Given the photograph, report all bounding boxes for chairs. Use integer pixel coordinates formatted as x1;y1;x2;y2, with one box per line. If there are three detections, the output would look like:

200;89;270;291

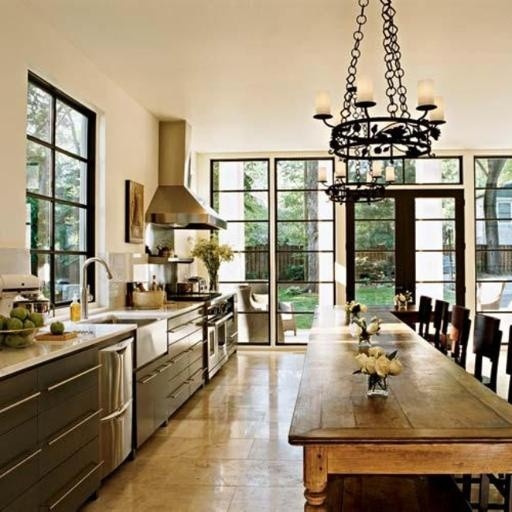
415;295;512;404
456;279;505;317
240;285;296;342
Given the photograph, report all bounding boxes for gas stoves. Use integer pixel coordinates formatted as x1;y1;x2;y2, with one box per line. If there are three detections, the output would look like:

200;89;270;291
166;291;222;301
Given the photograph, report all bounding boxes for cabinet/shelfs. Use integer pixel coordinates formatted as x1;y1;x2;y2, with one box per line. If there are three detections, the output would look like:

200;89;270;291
1;346;101;512
206;292;238;380
136;300;206;451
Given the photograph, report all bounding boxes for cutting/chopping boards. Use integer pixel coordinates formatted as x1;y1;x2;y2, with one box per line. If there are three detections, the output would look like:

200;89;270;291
35;333;78;341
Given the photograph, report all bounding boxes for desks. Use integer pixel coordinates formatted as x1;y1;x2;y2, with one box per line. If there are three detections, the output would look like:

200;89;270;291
291;302;512;511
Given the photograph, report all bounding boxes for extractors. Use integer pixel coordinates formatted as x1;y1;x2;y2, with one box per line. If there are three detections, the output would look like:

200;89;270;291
145;120;227;231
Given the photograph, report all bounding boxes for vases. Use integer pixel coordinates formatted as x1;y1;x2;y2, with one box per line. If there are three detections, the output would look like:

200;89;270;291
205;263;221;293
350;312;360;320
364;371;390;397
359;333;371;345
397;301;401;311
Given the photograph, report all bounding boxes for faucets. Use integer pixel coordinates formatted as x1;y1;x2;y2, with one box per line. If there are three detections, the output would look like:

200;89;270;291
81;257;113;319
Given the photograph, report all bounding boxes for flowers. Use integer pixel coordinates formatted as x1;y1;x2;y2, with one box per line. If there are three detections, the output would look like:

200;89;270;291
348;314;382;338
189;231;236;280
357;344;403;385
392;291;406;305
344;300;367;313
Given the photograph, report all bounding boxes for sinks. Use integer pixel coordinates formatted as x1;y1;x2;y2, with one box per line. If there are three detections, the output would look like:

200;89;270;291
74;313;158;331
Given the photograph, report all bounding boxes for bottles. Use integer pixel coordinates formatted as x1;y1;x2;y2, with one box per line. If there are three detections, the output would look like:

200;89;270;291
70;294;80;320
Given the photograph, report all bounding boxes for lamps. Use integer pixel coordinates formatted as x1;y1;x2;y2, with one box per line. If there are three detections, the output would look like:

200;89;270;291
313;0;447;205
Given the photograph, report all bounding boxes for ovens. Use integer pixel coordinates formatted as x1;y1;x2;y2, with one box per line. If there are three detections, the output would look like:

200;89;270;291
208;312;230;379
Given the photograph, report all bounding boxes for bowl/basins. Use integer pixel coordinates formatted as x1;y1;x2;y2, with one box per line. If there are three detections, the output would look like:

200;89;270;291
1;326;39;349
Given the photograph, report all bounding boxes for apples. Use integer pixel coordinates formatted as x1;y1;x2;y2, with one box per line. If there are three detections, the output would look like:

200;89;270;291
0;306;43;347
51;322;63;334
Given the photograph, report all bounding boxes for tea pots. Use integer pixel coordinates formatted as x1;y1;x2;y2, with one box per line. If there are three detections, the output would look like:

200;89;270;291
157;244;175;258
